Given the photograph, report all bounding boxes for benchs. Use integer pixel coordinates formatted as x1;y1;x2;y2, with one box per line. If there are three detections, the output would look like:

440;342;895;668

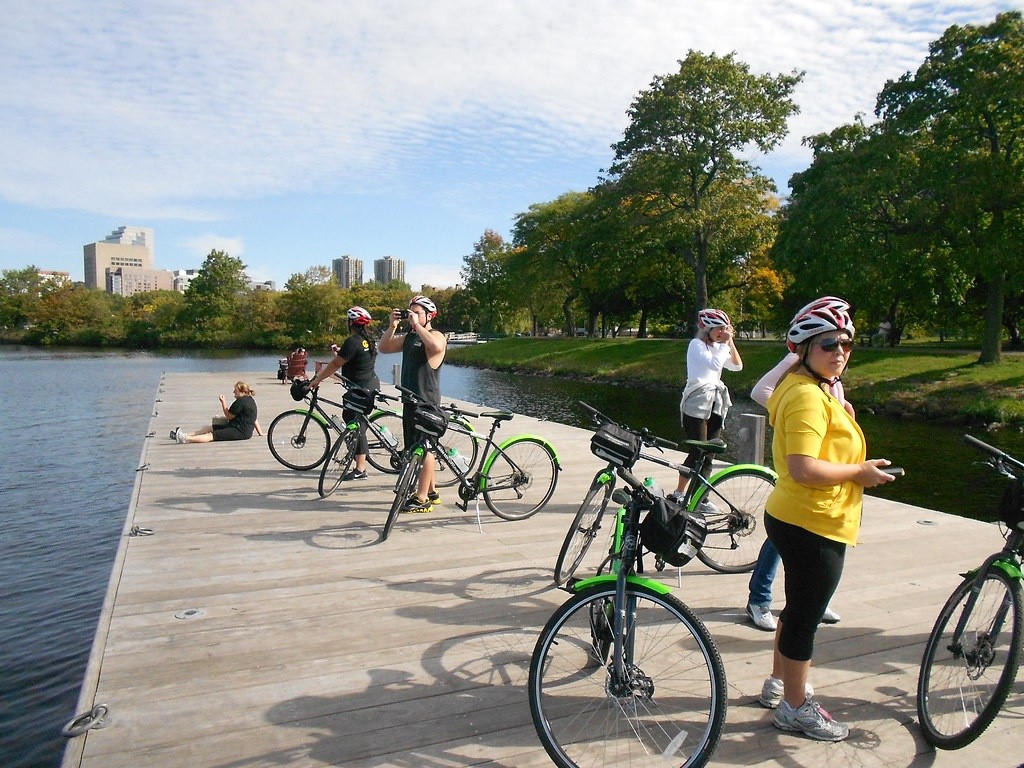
859;335;872;346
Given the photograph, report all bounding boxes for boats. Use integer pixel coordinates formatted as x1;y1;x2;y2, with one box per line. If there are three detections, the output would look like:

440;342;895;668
444;331;481;345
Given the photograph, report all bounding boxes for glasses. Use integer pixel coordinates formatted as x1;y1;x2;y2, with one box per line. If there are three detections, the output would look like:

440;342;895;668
809;337;854;354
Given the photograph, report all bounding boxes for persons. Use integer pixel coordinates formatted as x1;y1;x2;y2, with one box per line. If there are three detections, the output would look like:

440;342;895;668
673;309;743;513
305;306;380;481
170;382;267;443
759;307;895;741
745;296;855;630
872;317;891;347
379;295;446;513
293;348;308;364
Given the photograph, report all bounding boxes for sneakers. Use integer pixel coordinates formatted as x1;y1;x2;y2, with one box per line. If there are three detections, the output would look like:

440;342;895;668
773;695;851;742
408;488;441;505
744;602;777;631
758;674;815;710
169;430;177;440
399;495;434;514
341;467;368;481
675;488;687;506
821;608;841;626
698;500;724;515
175;426;187;444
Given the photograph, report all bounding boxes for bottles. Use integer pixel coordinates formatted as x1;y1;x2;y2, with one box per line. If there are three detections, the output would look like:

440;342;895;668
332;414;346;432
379;426;398;446
642;475;664;500
446;447;469;473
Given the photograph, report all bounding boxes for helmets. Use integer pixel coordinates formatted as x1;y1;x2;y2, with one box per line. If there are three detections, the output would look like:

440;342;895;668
408;295;437;318
786;295;854;326
345;305;373;326
697;308;731;329
786;306;856;354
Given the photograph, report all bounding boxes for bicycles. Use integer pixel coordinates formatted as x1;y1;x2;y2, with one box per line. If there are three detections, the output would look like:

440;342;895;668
380;384;563;542
267;372;408;475
527;463;729;768
916;433;1024;751
553;400;779;584
318;372;483;498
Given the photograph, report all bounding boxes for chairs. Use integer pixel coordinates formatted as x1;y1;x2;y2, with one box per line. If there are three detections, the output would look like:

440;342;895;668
283;354;309;384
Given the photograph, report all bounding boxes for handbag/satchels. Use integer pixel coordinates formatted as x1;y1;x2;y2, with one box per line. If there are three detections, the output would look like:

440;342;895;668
212;415;228;426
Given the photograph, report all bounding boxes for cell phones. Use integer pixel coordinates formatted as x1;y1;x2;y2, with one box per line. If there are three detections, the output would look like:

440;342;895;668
881;467;906;476
397;310;408;320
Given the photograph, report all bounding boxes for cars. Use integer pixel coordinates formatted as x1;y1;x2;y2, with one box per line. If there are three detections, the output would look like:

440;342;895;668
575;330;587;339
